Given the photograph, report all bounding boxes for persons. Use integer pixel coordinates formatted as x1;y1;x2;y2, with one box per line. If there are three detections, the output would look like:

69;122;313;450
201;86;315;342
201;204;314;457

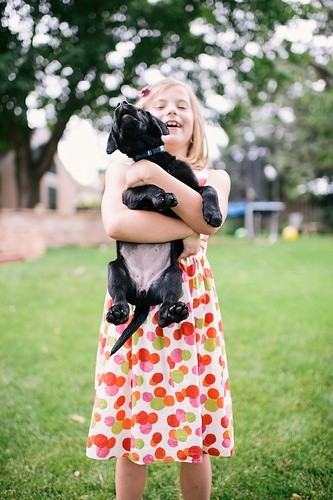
85;80;235;500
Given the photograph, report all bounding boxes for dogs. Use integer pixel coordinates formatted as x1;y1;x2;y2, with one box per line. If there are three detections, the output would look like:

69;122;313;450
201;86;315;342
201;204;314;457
106;100;223;358
